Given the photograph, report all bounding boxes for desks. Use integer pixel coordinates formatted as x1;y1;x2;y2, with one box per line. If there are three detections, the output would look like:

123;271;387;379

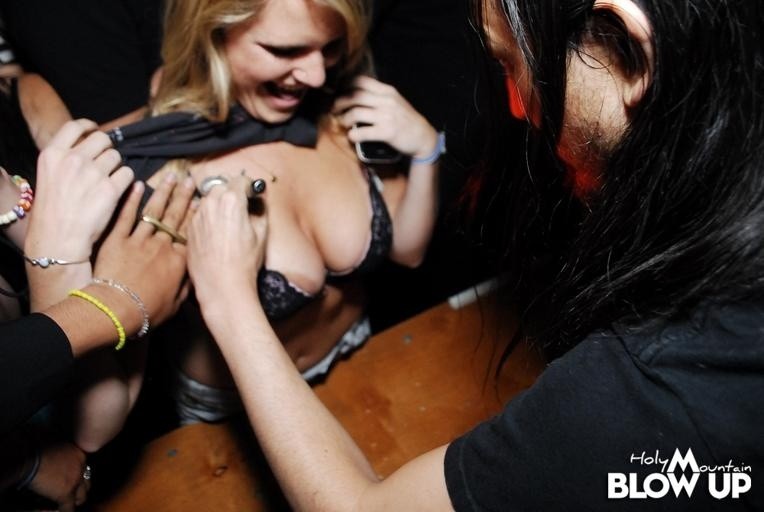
82;294;546;511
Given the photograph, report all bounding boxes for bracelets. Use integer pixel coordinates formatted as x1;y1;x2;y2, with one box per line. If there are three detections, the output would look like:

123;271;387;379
412;130;447;169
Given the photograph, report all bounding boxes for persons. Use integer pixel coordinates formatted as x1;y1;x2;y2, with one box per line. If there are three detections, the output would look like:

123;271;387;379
182;0;764;511
18;2;442;457
